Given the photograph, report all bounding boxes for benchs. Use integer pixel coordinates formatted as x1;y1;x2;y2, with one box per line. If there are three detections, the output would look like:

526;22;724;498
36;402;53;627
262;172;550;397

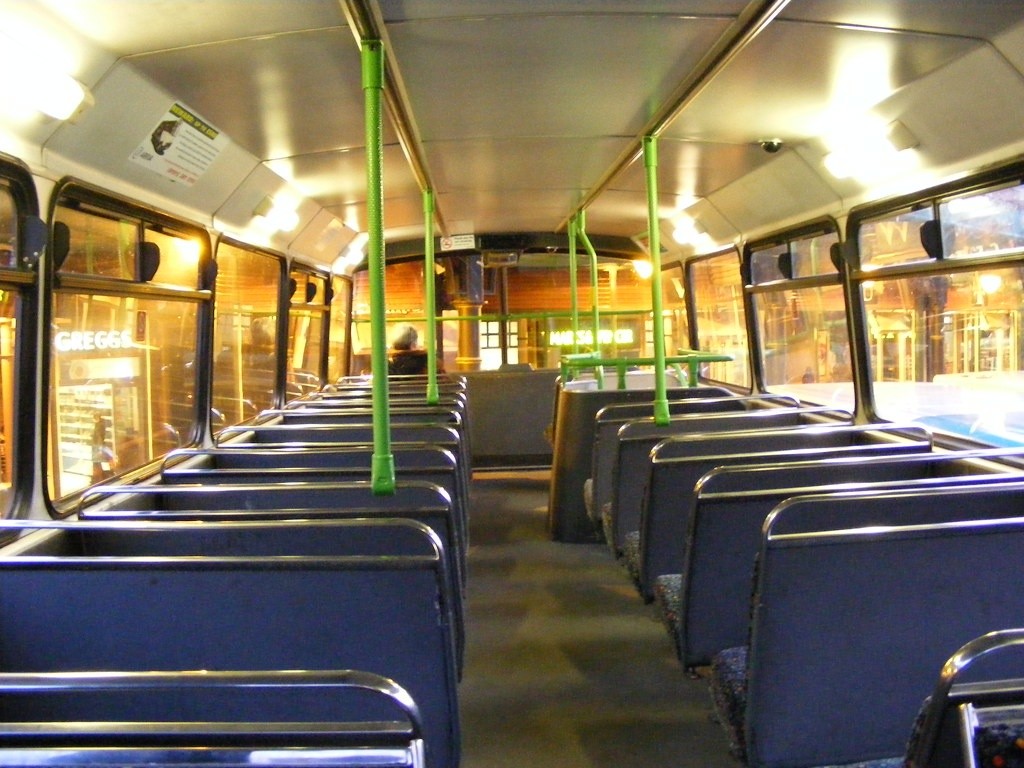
56;360;319;492
541;371;680;451
0;371;474;768
584;394;1024;768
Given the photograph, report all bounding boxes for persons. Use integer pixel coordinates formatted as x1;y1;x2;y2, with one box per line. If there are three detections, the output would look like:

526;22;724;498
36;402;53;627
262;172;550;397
387;323;445;375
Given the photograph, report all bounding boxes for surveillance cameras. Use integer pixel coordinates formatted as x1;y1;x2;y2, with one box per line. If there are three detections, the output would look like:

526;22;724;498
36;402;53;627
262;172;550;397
758;139;782;154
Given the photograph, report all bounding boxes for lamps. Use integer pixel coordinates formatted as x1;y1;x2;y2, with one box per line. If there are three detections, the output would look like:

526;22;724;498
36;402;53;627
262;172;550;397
37;79;95;127
255;196;300;232
821;118;921;180
